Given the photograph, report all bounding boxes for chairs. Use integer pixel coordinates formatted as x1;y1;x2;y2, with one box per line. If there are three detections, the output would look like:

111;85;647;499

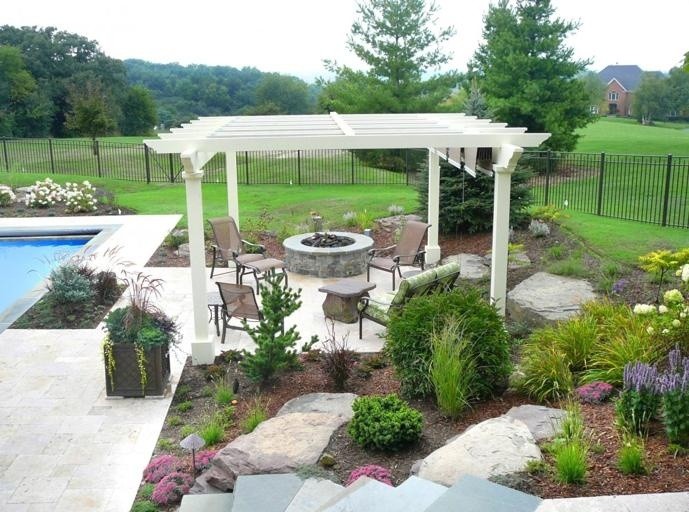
207;216;290;344
365;220;433;291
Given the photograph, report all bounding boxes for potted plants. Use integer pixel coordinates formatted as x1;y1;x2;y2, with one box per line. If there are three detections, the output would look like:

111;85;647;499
93;271;184;398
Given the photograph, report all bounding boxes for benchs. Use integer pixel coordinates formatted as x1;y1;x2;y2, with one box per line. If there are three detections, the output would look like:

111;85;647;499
356;263;460;339
